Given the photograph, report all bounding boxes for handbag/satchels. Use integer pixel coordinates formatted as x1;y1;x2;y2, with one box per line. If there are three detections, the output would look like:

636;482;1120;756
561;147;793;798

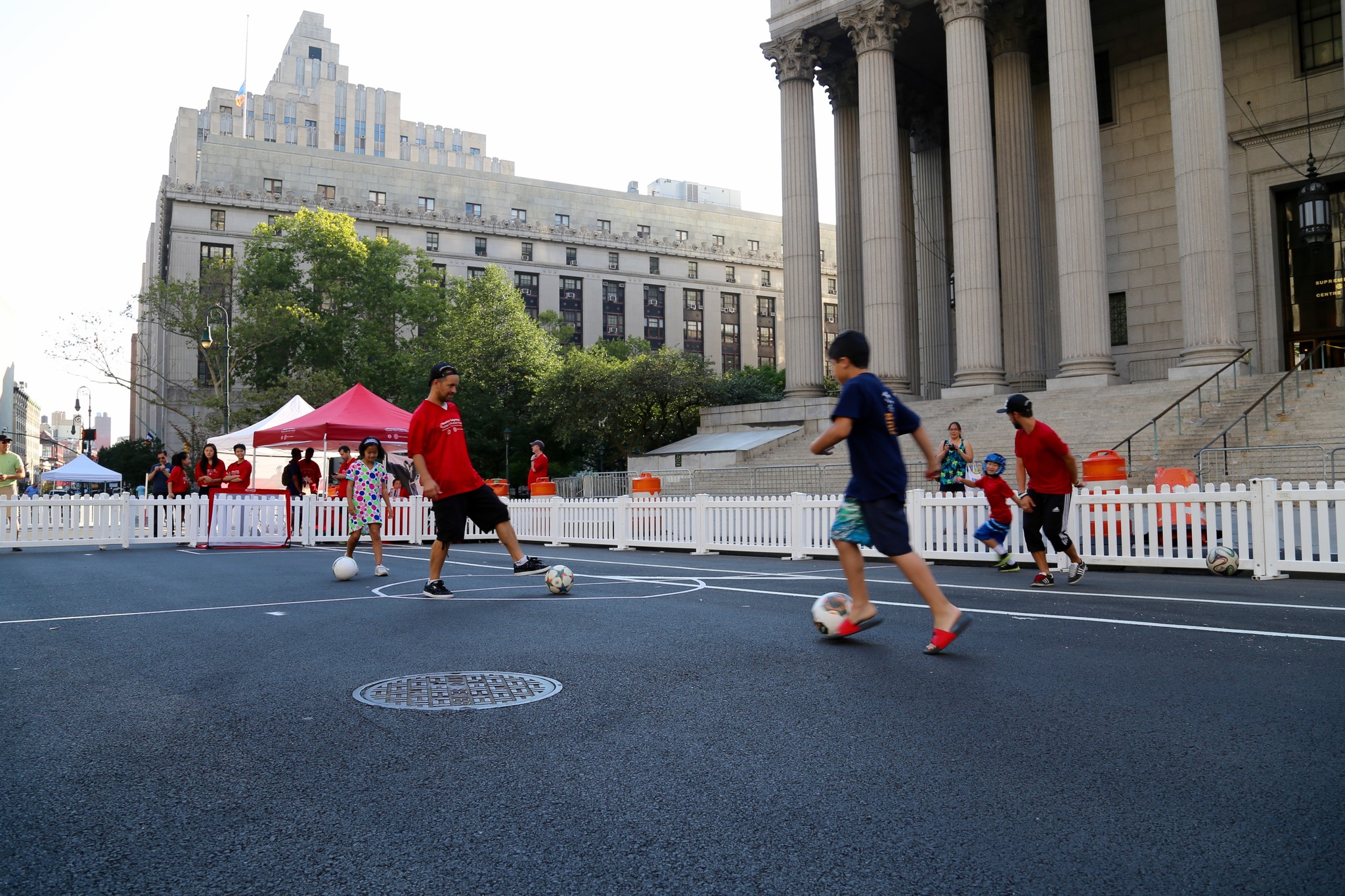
964;457;984;497
933;454;941;482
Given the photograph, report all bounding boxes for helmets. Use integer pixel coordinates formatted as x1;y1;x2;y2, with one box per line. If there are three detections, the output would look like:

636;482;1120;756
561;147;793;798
982;453;1006;476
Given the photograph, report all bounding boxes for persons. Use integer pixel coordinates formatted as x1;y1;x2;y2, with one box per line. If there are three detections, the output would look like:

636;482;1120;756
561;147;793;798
934;422;974;535
166;451;195;547
194;443;226;528
343;436;394;576
526;439;549;500
0;433;25;552
147;449;176;538
277;444;410;538
24;483;40;501
953;453;1023;572
135;481;146;499
405;360;556;599
807;330;976;655
222;444;252;537
994;394;1088;587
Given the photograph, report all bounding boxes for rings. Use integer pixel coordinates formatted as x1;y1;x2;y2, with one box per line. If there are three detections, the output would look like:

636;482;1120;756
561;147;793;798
1024;507;1027;508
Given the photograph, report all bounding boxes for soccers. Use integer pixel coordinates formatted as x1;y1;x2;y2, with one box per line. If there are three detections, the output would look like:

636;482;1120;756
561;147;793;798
332;556;359;580
810;591;853;635
544;564;574;595
1205;546;1240;577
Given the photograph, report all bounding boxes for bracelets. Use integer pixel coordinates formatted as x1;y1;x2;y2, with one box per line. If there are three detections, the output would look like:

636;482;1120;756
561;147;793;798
1071;479;1080;486
299;491;302;494
958;476;963;482
530;460;534;463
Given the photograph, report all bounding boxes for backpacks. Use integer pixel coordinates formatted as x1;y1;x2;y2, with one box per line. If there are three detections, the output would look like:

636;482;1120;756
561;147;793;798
282;462;297;486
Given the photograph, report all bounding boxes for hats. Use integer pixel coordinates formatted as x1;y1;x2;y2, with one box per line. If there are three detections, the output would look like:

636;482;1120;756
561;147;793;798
291;448;303;455
530;440;545;448
0;434;13;441
996;394;1032;413
428;362;457;386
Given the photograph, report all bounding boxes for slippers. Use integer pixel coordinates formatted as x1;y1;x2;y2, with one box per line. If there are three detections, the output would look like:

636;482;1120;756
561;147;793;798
924;614;973;654
833;616;885;639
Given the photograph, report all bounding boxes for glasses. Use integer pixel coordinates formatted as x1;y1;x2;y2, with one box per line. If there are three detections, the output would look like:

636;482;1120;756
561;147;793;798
949;429;960;432
34;485;37;487
0;441;10;444
158;456;166;460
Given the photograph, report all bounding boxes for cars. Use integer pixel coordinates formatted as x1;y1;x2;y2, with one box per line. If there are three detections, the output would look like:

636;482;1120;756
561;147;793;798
50;488;126;499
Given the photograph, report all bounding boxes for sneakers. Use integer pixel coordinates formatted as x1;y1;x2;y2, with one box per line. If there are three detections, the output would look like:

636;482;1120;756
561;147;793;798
1068;557;1086;585
1030;572;1054;587
997;563;1020;572
344;552;359;574
423;580;454;599
514;555;551;576
375;565;390;576
12;547;23;552
993;553;1014;566
176;542;190;546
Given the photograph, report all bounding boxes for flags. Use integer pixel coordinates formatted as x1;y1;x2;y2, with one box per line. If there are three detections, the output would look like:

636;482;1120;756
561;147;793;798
235;81;245;107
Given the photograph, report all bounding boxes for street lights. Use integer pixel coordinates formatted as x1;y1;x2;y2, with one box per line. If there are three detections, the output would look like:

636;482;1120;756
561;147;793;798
201;301;231;434
598;446;604;473
71;413;85;455
74;386;92;461
502;427;510;478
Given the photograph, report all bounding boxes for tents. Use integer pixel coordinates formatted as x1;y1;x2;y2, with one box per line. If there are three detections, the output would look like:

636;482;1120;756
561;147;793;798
205;381;425;496
40;453;122;498
